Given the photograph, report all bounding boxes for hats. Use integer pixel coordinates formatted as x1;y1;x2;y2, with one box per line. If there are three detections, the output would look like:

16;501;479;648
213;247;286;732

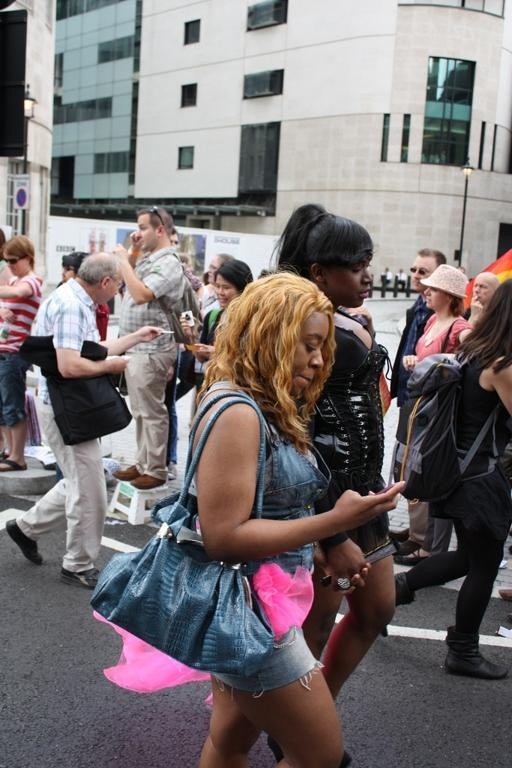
419;264;469;299
62;251;90;267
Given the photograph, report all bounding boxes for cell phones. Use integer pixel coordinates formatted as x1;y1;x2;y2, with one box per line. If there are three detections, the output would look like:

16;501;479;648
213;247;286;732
372;480;407;495
182;311;195;327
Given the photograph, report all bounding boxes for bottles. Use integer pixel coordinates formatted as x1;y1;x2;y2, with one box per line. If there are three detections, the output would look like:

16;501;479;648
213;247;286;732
0;319;12;344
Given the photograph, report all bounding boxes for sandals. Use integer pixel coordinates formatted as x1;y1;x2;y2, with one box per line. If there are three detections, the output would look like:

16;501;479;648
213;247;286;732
393;547;429;566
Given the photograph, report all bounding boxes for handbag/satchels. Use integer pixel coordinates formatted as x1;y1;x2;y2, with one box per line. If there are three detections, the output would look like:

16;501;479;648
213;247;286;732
88;389;271;682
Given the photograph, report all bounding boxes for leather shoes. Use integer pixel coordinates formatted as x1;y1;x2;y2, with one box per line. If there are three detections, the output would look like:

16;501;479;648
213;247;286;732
130;473;166;490
389;528;423;556
112;465;141;482
59;564;102;591
5;518;44;564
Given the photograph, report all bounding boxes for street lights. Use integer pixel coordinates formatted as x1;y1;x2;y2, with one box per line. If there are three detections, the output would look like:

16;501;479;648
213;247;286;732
456;157;476;268
21;83;38;235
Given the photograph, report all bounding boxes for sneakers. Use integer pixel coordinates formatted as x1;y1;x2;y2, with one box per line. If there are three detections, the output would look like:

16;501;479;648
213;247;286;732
167;465;178;480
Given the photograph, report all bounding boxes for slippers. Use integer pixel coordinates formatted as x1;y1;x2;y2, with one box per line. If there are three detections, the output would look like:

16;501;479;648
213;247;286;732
0;448;27;472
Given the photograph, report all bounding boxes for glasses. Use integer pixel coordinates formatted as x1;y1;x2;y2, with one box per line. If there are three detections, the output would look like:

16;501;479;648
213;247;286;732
149;205;165;226
409;266;433;274
66;266;75;271
3;255;28;264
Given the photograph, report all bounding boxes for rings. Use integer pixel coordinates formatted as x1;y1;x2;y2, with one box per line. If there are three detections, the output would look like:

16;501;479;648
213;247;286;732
337;577;350;589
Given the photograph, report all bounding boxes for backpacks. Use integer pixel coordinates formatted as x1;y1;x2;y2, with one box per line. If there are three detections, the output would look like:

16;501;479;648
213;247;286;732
391;348;505;506
151;251;204;345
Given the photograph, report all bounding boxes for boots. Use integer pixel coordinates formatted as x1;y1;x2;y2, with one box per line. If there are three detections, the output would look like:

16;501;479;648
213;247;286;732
443;625;508;680
378;570;417;638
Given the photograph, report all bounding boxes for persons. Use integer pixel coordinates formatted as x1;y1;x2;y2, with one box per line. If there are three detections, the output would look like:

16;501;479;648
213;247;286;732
0;205;253;590
382;249;512;678
267;204;396;768
189;270;407;767
